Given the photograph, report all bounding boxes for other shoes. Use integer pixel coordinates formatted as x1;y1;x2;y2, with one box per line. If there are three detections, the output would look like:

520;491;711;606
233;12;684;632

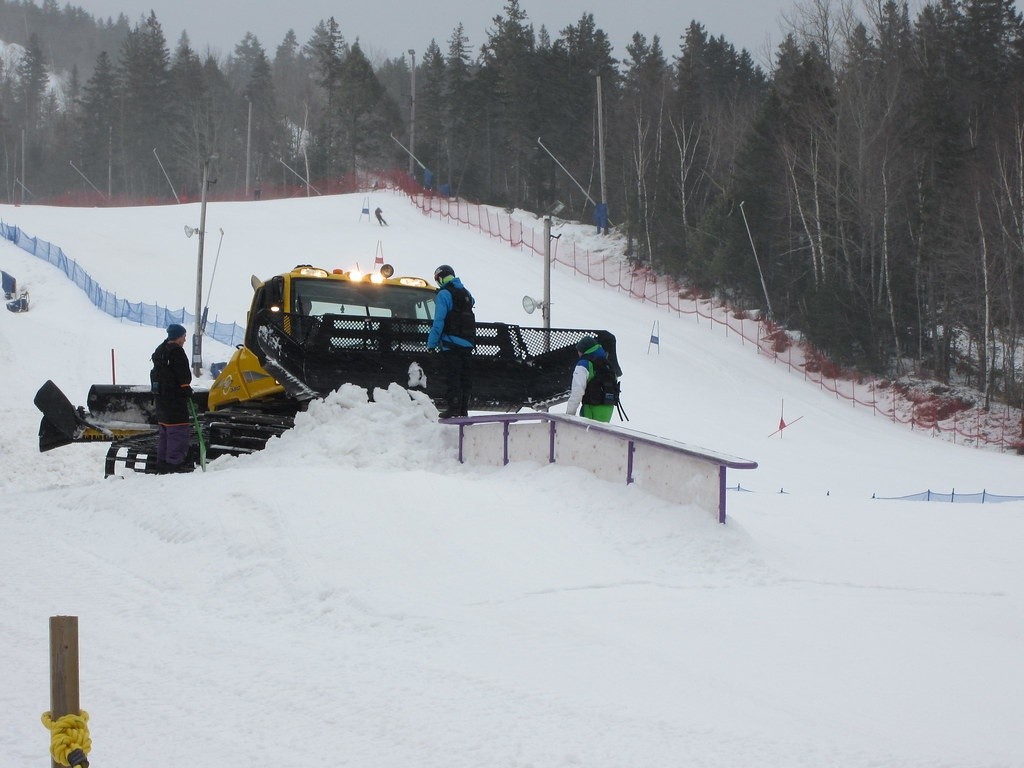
156;462;194;474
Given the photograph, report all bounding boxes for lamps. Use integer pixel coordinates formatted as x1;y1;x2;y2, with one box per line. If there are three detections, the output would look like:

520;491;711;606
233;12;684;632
522;295;543;315
184;224;199;238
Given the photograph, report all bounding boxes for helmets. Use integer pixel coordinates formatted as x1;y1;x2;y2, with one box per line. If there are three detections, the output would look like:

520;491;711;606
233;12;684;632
434;265;455;282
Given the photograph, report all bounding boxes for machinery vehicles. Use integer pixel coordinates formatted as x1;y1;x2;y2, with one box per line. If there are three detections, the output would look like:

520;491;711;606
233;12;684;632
34;264;622;475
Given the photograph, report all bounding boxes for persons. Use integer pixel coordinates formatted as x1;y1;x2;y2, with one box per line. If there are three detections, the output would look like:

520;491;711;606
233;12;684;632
375;208;388;225
153;324;195;473
255;177;261;200
427;265;475;418
565;337;614;422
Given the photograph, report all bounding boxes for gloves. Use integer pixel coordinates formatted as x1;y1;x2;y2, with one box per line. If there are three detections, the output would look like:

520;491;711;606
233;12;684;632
182;386;193;400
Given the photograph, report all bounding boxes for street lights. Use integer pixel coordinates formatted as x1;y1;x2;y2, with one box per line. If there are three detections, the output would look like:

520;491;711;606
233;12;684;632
244;94;253;199
589;67;610;227
184;159;218;374
407;48;416;176
520;219;561;352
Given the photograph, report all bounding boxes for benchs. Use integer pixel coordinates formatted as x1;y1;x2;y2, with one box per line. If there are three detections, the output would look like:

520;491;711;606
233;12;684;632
438;414;758;524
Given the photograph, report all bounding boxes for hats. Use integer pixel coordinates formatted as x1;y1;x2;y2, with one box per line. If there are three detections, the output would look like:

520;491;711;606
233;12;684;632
576;337;597;354
167;324;186;340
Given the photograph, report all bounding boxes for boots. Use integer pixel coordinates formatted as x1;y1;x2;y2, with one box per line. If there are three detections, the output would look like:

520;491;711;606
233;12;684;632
438;393;460;418
460;392;472;417
